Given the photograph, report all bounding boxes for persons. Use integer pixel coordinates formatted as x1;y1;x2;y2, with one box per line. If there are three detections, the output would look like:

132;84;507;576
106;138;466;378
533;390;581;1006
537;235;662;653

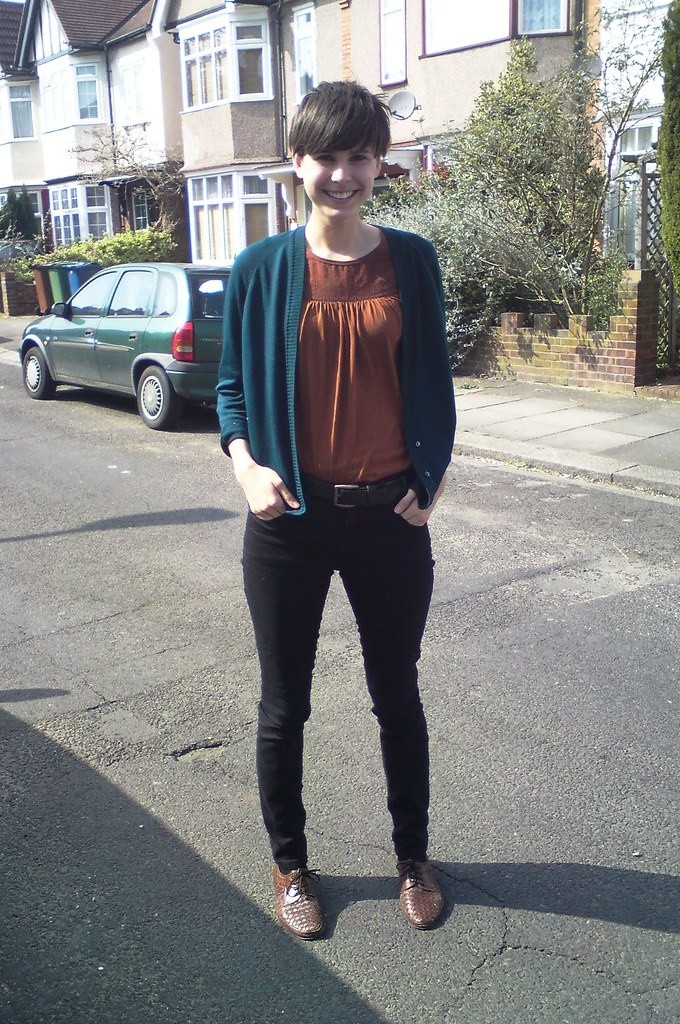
217;81;456;936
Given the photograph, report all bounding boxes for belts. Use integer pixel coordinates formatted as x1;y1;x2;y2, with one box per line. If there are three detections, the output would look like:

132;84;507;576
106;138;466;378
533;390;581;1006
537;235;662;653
301;472;415;509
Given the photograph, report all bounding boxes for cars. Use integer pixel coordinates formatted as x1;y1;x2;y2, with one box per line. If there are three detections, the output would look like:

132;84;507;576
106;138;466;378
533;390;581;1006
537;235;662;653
15;259;236;432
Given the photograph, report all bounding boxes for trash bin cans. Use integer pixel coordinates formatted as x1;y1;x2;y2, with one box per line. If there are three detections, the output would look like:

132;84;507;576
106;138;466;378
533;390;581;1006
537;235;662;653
42;260;78;304
27;262;54;316
61;262;103;298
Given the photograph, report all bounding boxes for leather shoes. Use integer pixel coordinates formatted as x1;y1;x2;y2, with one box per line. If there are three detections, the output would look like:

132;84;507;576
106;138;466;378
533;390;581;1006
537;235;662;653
396;855;445;930
273;863;325;941
